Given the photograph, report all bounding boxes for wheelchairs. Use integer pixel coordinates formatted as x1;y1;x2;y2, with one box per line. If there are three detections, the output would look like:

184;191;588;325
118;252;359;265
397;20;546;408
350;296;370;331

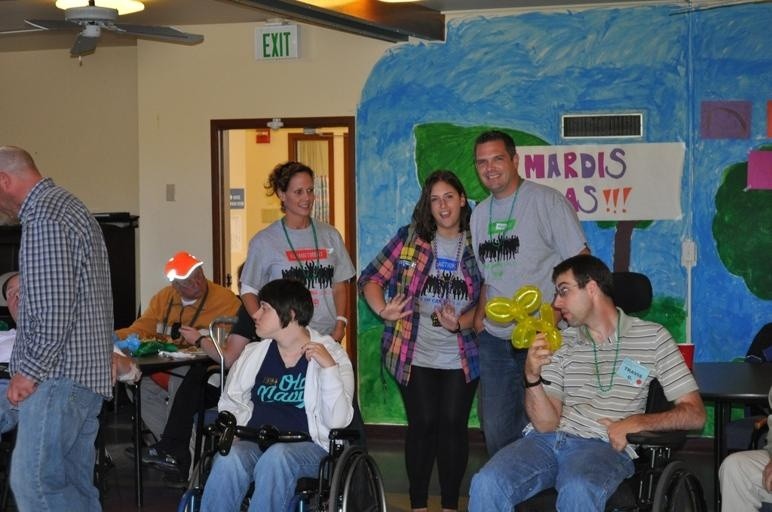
125;363;228;473
179;317;388;511
516;271;707;511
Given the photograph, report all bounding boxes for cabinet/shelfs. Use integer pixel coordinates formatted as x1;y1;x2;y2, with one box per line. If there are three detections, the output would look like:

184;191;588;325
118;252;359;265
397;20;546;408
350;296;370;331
0;224;135;331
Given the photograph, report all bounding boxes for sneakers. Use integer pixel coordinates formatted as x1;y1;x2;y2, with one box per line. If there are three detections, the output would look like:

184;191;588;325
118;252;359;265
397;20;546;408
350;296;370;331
124;444;180;473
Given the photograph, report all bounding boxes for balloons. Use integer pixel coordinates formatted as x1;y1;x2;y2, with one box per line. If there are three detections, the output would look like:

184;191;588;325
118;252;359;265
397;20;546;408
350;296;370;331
485;285;564;353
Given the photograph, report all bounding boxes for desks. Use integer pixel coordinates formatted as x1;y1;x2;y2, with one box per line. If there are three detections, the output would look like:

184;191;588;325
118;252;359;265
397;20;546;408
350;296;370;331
687;361;772;512
129;345;217;512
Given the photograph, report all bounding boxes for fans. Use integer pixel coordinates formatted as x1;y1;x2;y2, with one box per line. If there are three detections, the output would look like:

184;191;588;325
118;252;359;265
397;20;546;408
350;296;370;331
0;0;204;59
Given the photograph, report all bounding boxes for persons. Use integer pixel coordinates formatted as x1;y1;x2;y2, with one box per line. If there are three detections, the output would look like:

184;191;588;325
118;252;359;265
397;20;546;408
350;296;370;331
468;130;597;459
122;265;262;474
464;254;708;512
239;158;357;342
194;281;359;512
1;271;147;446
114;252;245;461
716;386;772;512
361;169;485;512
1;147;113;511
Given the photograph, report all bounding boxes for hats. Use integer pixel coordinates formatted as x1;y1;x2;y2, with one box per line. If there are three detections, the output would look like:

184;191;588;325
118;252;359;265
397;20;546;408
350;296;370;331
163;250;204;281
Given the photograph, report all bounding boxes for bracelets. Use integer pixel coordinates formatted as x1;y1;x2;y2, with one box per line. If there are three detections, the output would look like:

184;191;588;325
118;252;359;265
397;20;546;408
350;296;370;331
334;315;348;327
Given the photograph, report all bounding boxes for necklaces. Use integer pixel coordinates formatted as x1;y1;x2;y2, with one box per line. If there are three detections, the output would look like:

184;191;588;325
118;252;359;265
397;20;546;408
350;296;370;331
280;217;320;281
487;182;522;248
432;224;465;310
584;310;620;393
